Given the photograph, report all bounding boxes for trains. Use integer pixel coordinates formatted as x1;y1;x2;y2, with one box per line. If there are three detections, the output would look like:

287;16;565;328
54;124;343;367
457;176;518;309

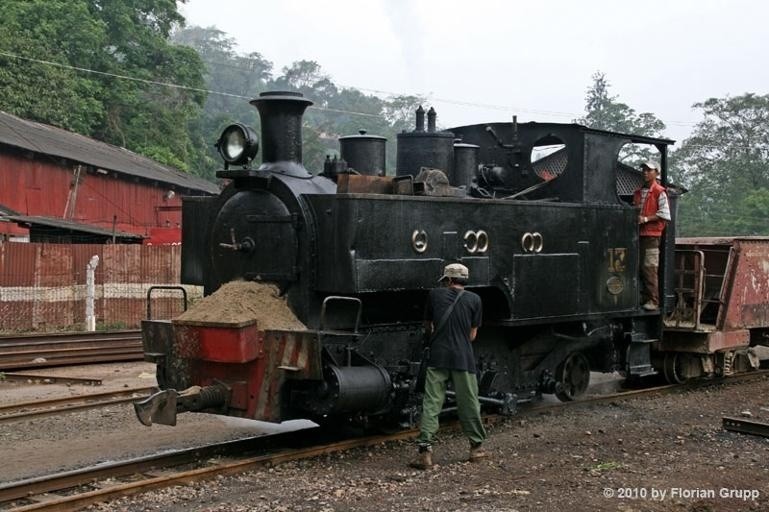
133;90;769;434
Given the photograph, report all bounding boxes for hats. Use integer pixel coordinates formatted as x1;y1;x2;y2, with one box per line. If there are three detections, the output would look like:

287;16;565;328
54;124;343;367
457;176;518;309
435;264;469;284
639;159;660;174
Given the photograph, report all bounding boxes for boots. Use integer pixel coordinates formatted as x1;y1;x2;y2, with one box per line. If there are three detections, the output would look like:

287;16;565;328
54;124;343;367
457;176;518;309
409;443;434;468
469;448;491;463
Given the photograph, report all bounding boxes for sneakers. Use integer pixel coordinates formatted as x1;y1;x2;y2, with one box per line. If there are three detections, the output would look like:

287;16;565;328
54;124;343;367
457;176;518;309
642;300;659;311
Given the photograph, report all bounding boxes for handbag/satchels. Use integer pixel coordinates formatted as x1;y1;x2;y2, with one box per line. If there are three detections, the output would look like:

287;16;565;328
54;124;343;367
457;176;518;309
413;352;429;394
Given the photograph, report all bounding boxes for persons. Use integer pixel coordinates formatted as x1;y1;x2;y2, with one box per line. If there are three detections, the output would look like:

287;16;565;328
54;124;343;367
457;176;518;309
633;161;671;311
410;263;493;469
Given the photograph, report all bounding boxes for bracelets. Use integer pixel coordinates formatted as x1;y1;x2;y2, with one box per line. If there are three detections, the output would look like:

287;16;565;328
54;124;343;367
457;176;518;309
645;217;648;223
